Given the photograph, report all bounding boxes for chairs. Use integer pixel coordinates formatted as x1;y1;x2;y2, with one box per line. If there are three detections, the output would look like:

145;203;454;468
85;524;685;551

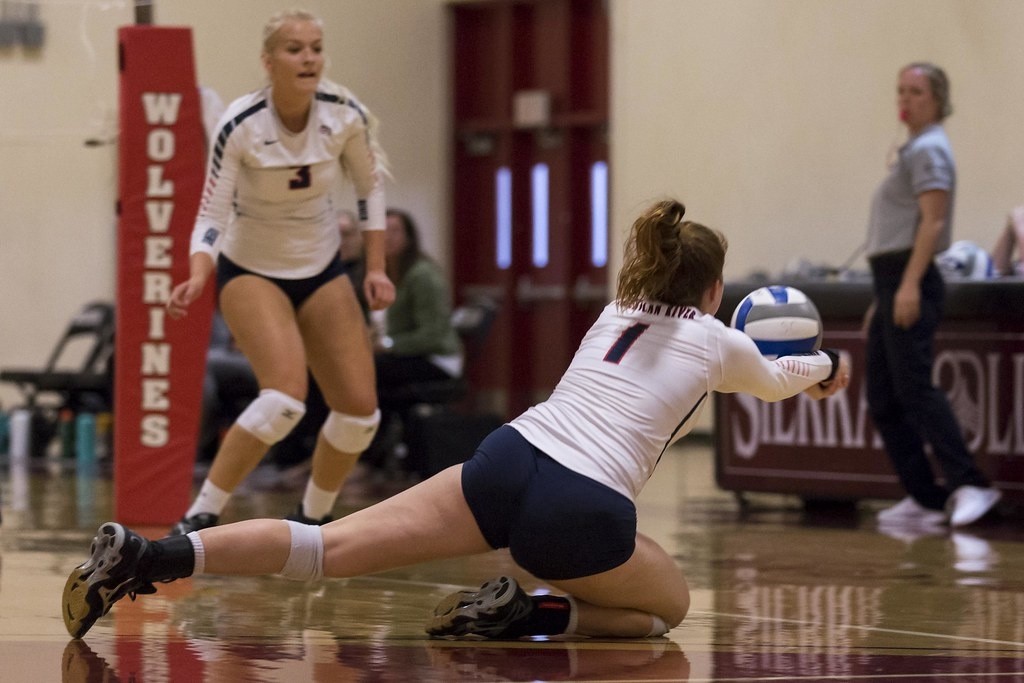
0;303;113;459
409;305;495;413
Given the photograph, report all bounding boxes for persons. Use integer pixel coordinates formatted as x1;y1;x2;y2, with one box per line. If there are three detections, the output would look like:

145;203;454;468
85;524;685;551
167;11;463;541
63;201;851;639
62;636;689;683
860;63;1001;525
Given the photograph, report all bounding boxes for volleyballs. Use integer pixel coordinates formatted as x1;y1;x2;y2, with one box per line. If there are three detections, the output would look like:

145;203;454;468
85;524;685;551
730;283;827;361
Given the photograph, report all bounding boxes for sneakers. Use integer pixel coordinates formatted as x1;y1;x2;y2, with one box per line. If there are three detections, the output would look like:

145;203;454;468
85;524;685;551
950;484;1001;524
283;503;332;526
62;522;148;639
879;497;947;527
163;513;219;538
425;577;532;638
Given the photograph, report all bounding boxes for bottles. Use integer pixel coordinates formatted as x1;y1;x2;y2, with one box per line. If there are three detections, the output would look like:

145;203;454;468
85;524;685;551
6;404;111;461
